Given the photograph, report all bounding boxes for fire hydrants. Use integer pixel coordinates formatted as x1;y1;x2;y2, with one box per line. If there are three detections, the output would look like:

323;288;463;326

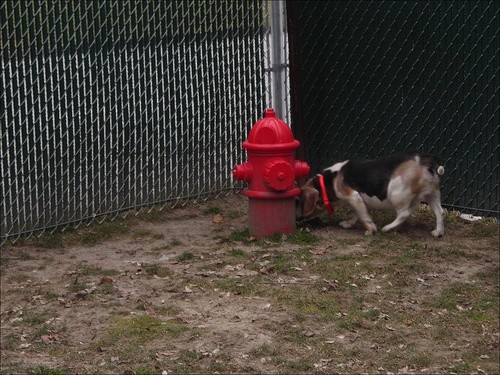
232;108;310;236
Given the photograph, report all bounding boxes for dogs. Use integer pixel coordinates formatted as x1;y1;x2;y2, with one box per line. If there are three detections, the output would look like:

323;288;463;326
297;153;447;238
